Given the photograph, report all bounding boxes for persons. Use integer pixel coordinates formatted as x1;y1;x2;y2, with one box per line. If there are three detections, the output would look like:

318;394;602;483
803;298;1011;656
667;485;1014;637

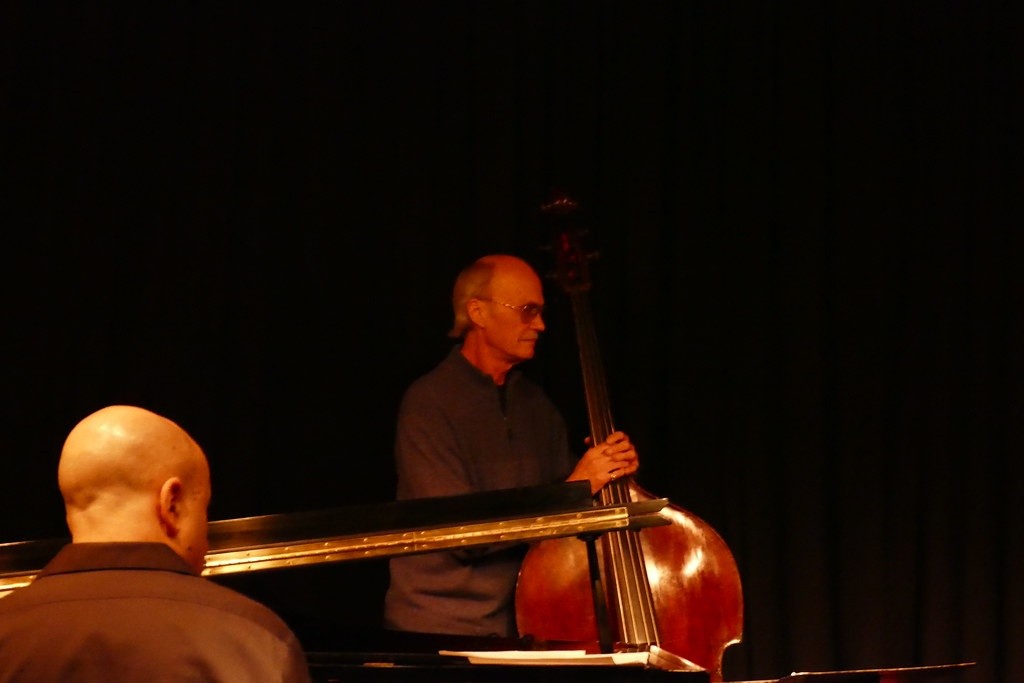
381;254;641;638
0;405;315;683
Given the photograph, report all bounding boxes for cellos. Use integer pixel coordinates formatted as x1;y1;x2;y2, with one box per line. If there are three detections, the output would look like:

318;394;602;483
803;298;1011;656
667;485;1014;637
514;227;745;683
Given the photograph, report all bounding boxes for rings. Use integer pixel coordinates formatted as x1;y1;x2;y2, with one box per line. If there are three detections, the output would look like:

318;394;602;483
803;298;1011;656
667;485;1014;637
610;472;616;481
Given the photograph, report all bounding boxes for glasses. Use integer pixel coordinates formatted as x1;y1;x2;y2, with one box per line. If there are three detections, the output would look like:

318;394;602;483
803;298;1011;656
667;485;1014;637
470;298;546;325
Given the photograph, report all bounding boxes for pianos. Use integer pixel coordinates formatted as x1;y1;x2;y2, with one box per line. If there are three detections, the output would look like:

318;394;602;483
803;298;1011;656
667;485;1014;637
0;478;712;682
